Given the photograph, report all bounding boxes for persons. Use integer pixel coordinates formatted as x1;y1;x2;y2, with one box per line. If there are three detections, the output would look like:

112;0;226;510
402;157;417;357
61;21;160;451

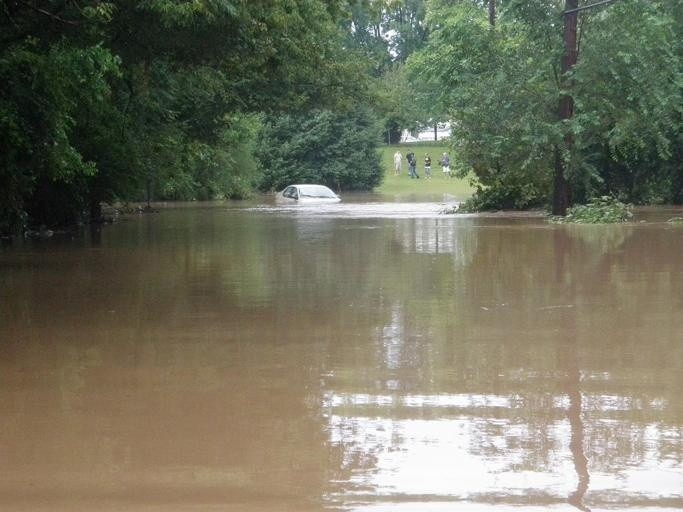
392;149;403;175
423;152;431;179
404;149;414;176
440;152;451;179
409;153;419;179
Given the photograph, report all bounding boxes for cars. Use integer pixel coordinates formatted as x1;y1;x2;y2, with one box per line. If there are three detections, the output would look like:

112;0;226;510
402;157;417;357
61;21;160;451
274;183;342;203
